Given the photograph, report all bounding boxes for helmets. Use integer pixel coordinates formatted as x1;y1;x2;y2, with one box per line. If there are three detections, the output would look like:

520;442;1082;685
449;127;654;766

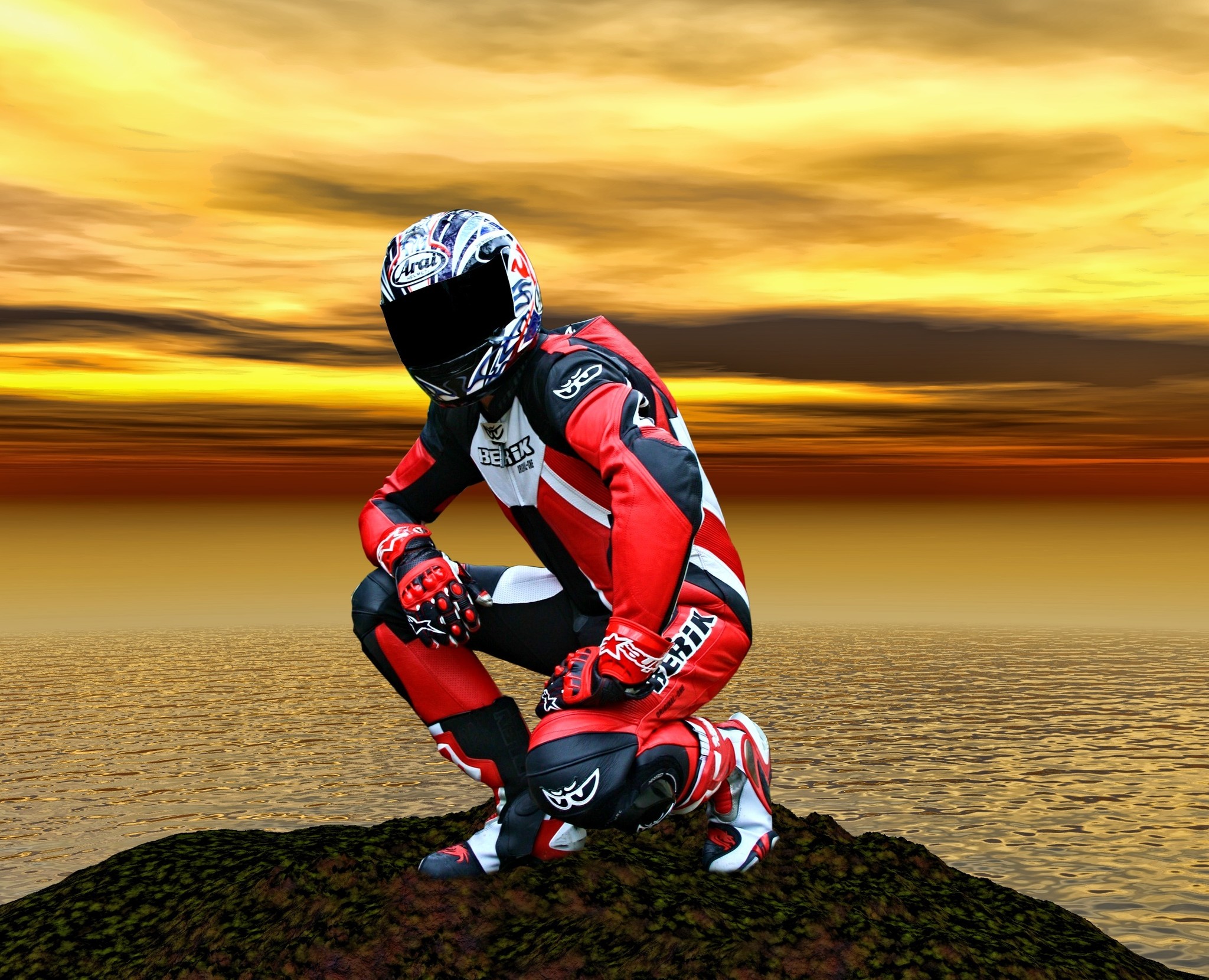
378;209;542;408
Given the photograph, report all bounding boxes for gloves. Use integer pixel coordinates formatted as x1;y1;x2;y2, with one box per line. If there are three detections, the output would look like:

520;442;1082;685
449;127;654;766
534;617;676;718
372;524;494;649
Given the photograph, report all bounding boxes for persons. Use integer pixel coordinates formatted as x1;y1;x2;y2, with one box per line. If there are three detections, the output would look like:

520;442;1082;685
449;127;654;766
349;208;777;877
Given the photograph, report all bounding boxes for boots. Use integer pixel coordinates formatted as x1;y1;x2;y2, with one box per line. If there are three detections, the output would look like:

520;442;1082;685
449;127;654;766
416;692;588;880
681;711;779;874
